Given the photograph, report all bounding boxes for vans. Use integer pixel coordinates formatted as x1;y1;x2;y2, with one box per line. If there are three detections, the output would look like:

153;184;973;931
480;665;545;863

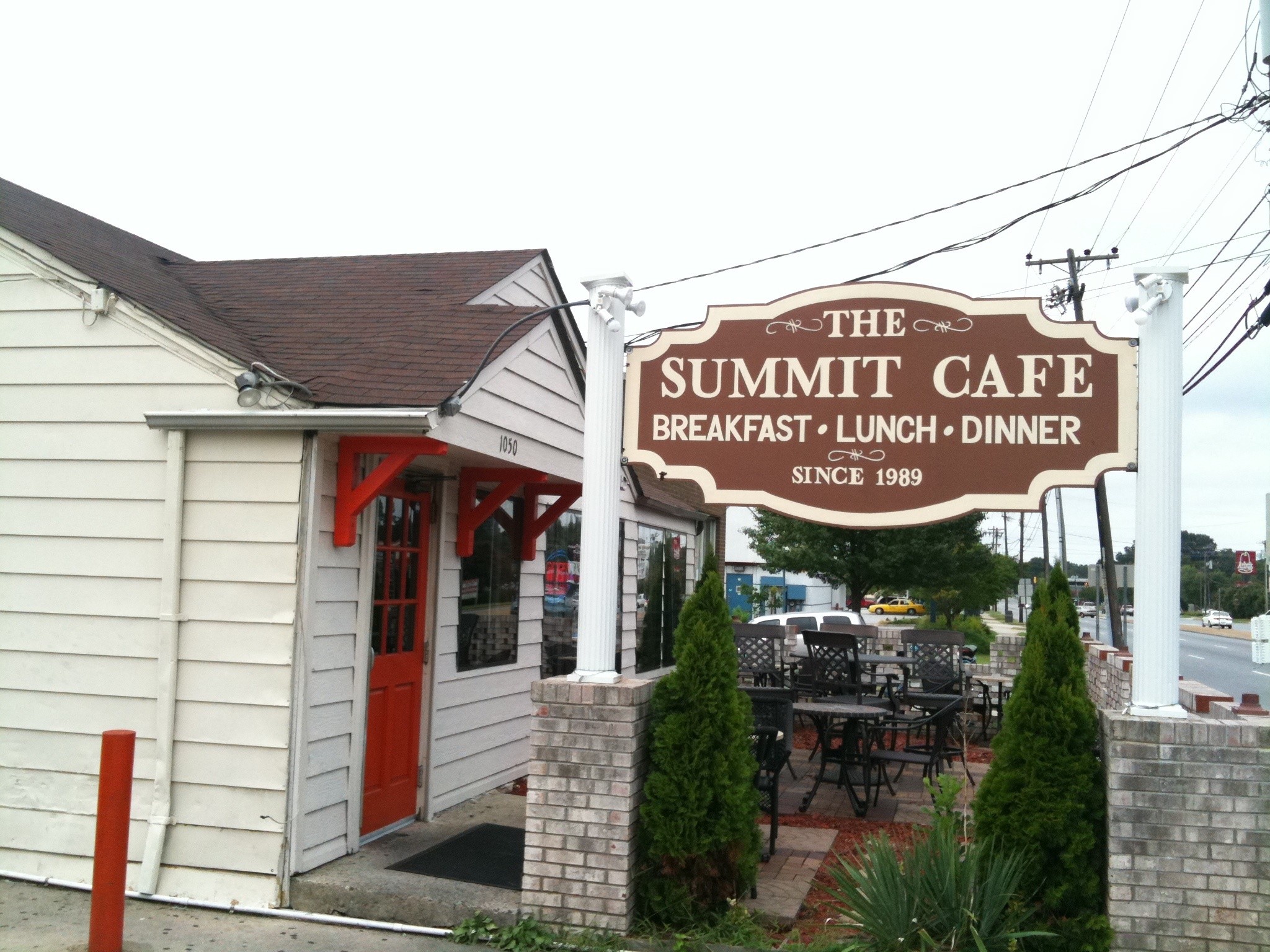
877;596;907;604
736;610;870;676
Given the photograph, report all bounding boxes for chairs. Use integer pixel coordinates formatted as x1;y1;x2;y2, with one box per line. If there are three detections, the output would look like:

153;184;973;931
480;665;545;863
749;696;798;782
745;725;779;856
892;629;992;819
732;623;800;687
458;612;479;671
737;686;796;703
801;623;899;762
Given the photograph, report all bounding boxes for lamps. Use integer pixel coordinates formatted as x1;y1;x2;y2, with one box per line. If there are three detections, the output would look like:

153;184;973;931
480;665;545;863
1124;273;1173;325
235;374;262;408
590;282;633;333
626;299;645;317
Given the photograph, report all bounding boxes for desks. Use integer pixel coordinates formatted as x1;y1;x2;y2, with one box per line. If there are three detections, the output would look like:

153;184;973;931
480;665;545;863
962;673;1017;744
747;729;784;786
790;650;919;694
813;696;890;786
791;701;890;818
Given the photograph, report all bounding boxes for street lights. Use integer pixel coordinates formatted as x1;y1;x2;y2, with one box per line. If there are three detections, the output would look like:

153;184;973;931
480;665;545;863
565;273;645;686
1121;267;1189;720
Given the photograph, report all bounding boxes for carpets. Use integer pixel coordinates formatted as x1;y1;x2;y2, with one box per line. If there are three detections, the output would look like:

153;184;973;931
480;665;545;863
383;821;527;892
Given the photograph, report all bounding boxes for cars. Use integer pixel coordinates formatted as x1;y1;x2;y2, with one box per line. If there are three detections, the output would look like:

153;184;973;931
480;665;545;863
1083;602;1096;610
1075;606;1096;618
1120;605;1133;616
1201;608;1218;614
845;593;875;609
868;599;926;616
1201;611;1232;629
1259;609;1270;618
